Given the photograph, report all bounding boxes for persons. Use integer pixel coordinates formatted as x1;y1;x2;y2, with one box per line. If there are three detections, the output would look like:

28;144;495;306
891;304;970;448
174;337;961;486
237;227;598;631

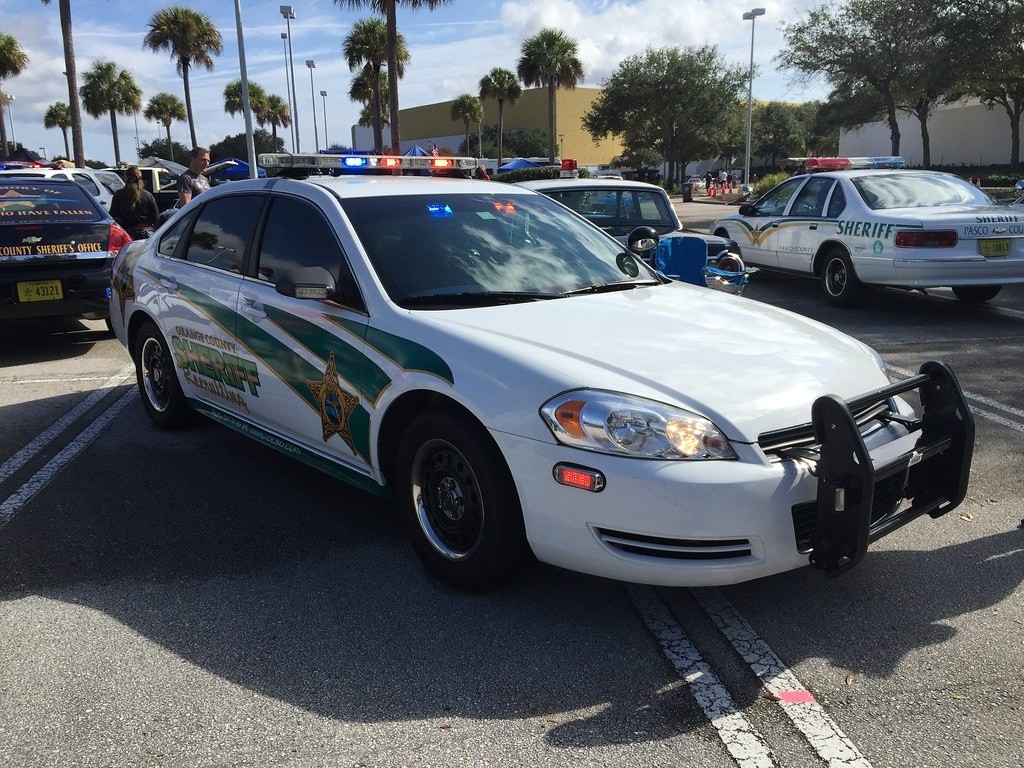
719;170;728;188
706;170;712;193
732;172;759;184
258;267;276;282
475;164;490;181
177;147;213;208
107;165;161;240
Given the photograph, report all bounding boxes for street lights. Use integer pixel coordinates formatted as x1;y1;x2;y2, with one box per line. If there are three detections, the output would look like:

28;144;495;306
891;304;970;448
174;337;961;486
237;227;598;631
280;32;296;153
305;59;319;153
741;8;768;195
279;5;302;153
320;90;330;151
5;93;19;151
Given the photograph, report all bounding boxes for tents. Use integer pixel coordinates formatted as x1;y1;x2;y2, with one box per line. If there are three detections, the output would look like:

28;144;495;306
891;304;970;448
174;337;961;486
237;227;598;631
401;146;437;177
128;156;191;188
0;147;93;170
205;157;267;187
496;158;543;174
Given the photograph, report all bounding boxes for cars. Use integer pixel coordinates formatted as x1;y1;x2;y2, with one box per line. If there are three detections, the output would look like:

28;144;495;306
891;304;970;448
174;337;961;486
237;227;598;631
0;167;134;334
492;159;747;297
710;155;1024;307
105;151;979;602
